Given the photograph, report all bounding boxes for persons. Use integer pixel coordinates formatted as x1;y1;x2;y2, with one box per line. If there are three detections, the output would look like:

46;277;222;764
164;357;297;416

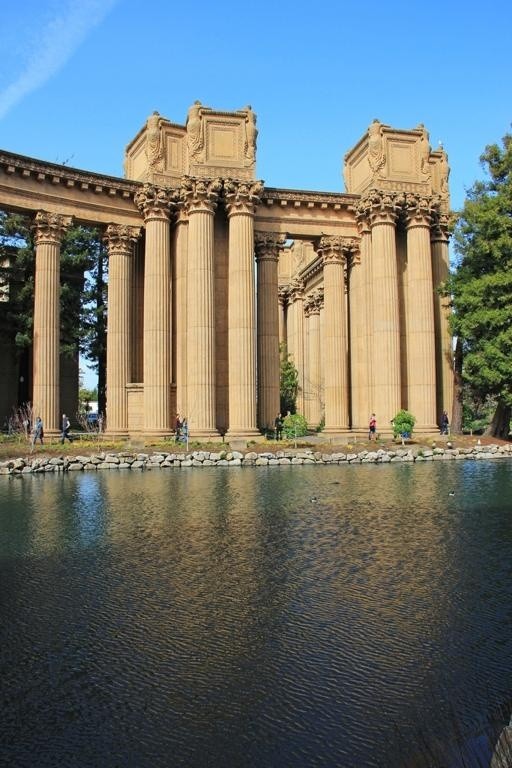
369;412;376;440
440;410;450;434
171;413;182;441
61;412;73;446
179;417;189;441
273;412;284;441
284;410;292;420
32;416;43;447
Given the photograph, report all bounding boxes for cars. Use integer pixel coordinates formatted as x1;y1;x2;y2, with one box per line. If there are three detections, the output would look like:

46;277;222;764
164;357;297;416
85;414;99;427
